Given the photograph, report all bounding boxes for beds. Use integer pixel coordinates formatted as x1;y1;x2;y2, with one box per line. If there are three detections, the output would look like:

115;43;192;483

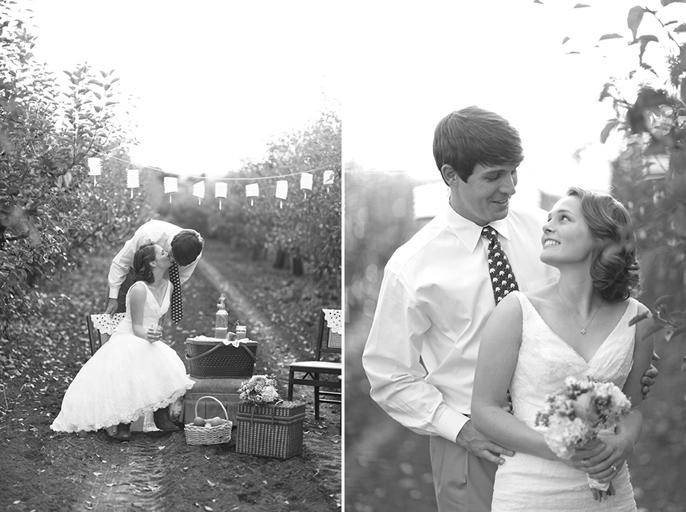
180;374;250;424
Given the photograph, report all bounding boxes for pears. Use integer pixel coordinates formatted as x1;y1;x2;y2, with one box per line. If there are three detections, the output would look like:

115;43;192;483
194;417;206;425
210;417;225;427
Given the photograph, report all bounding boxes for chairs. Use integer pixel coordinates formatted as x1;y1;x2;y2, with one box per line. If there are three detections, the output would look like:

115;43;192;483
88;312;125;358
286;307;341;436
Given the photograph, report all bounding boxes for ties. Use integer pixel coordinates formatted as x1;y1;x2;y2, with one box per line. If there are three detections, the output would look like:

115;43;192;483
481;226;518;306
169;264;184;325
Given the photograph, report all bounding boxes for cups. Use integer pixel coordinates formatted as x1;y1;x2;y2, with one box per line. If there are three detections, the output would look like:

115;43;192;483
235;326;247;340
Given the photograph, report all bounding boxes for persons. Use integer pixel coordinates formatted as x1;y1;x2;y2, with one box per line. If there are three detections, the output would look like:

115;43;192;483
50;218;206;440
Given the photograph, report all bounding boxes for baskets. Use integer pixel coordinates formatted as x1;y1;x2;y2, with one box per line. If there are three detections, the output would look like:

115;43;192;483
184;338;258;379
235;400;305;460
184;396;233;446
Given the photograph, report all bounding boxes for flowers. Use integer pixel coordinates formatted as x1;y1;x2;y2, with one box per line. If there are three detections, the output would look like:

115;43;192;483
237;369;282;404
530;370;631;501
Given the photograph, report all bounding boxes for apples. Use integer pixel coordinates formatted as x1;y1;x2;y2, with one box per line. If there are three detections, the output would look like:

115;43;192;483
228;331;238;341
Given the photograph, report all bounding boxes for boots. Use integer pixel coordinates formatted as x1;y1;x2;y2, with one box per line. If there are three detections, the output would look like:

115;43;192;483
155;408;180;431
116;423;132;440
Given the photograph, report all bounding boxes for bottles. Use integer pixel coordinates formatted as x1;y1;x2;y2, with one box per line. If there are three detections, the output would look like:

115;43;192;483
215;304;229;339
